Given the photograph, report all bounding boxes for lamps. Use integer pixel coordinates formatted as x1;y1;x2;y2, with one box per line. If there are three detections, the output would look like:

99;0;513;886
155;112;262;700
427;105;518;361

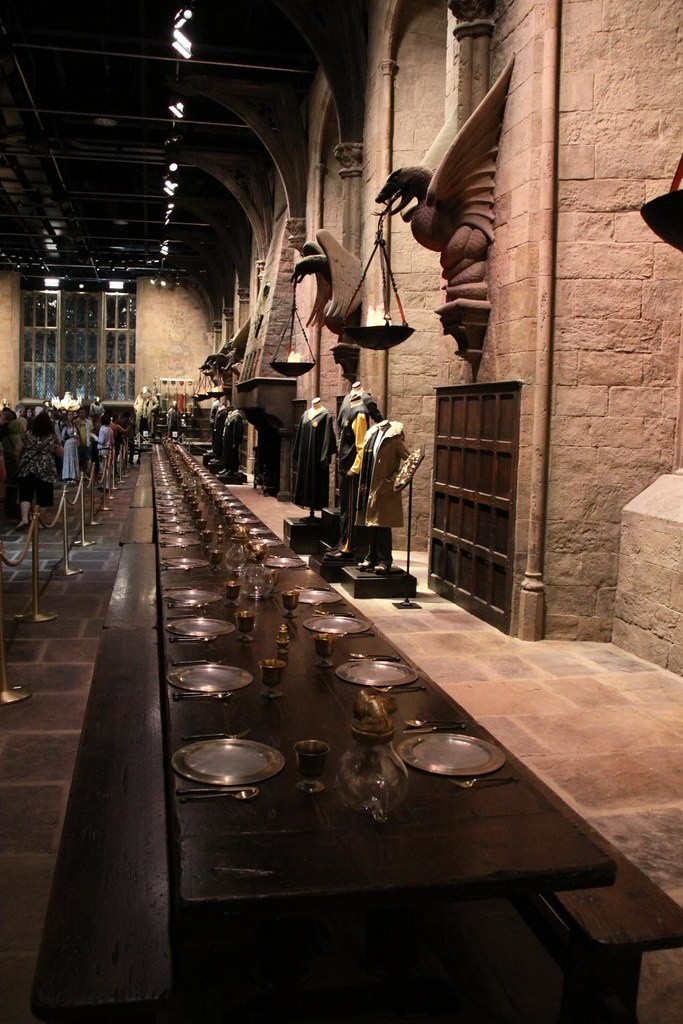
171;9;200;56
149;275;159;284
160;276;170;286
167;149;179;172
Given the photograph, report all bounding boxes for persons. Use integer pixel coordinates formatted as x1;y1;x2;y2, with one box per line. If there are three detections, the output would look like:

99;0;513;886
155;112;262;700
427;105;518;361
61;391;73;405
90;397;105;428
207;400;242;476
326;382;384;562
355;420;405;572
166;402;180;438
289;398;335;511
132;386;159;435
0;398;136;531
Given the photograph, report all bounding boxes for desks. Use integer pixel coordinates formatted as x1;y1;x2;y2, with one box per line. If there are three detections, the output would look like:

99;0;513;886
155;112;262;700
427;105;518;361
149;441;617;923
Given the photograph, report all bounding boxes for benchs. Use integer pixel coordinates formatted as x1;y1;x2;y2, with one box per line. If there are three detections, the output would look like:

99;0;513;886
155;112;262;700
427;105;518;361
31;454;179;1024
471;722;683;1024
